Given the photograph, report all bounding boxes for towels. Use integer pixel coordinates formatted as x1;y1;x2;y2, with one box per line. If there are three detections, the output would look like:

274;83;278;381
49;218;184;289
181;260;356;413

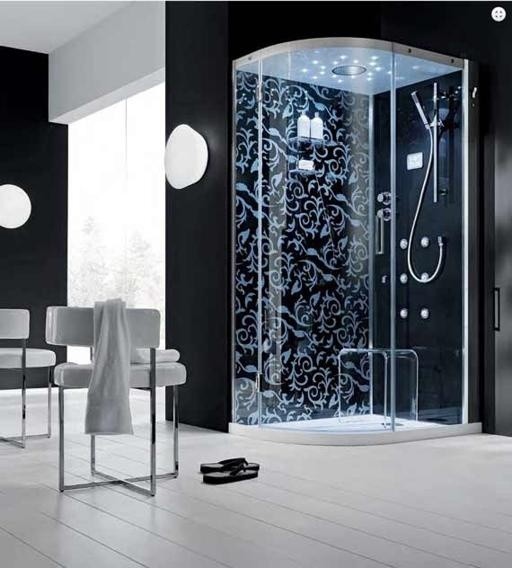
85;298;180;434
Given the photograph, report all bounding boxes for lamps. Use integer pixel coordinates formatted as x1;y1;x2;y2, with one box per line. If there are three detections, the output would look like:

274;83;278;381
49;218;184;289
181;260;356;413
164;123;209;190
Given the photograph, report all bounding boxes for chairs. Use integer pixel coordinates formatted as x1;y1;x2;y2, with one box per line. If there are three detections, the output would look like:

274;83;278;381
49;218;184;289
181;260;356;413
46;306;188;498
0;307;56;448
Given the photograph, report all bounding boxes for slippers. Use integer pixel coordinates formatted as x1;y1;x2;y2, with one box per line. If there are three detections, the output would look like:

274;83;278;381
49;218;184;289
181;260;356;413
200;457;259;472
203;461;258;484
